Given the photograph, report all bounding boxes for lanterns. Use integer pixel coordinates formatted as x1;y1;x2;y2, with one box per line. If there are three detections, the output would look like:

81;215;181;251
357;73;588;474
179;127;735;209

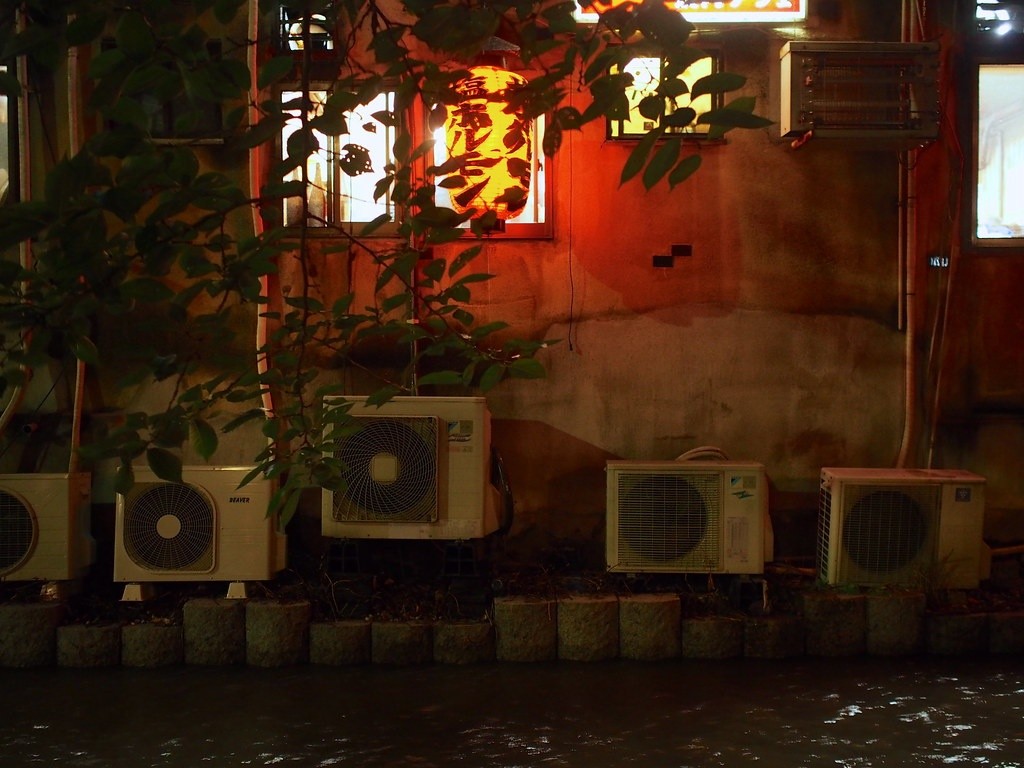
444;63;534;232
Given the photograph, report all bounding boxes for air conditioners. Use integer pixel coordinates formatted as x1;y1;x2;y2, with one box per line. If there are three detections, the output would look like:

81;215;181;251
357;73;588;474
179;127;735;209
114;465;289;600
816;468;992;592
322;396;506;539
605;459;775;575
0;472;97;582
